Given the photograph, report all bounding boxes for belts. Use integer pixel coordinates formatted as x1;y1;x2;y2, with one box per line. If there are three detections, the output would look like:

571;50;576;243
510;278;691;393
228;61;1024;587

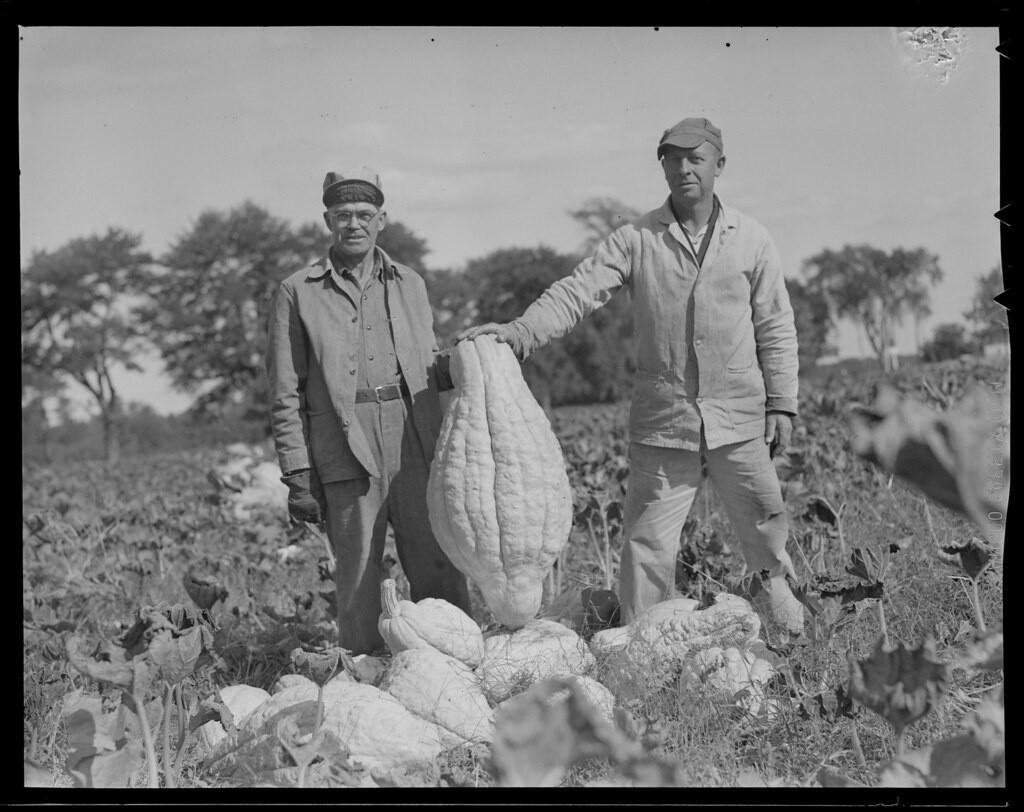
354;383;409;404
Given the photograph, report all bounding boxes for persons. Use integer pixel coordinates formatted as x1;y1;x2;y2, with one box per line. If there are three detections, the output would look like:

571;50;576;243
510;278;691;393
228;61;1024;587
270;171;456;660
455;117;807;634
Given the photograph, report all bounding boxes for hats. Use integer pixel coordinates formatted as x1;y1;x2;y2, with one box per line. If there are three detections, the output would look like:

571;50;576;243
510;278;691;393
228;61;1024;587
323;169;385;205
656;118;723;160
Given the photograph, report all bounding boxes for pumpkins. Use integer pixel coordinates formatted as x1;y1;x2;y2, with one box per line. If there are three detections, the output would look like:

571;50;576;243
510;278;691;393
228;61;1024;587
187;580;786;787
424;333;574;630
210;444;291;523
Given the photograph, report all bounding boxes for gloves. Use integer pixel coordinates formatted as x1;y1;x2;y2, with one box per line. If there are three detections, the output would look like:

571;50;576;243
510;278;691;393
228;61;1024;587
281;472;327;524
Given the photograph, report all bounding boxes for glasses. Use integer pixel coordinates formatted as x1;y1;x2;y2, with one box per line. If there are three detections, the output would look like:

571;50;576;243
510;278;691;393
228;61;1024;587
328;209;382;229
